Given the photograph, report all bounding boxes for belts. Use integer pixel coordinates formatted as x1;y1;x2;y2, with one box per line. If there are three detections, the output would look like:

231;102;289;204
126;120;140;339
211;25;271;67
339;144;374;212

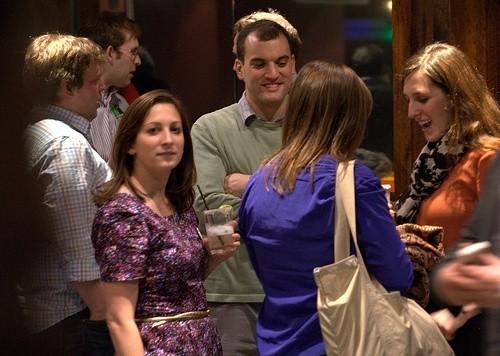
134;310;209;328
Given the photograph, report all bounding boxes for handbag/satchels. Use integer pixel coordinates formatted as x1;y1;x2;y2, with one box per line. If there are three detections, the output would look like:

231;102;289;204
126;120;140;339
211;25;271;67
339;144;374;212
313;159;456;356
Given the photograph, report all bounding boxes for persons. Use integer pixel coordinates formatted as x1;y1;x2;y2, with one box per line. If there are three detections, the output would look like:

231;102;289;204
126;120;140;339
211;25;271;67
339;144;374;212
190;10;302;356
388;41;499;356
91;89;242;356
0;12;143;356
239;62;414;356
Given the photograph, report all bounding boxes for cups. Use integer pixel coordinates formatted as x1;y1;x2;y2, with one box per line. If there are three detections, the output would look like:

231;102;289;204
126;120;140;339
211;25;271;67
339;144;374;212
204;207;235;256
